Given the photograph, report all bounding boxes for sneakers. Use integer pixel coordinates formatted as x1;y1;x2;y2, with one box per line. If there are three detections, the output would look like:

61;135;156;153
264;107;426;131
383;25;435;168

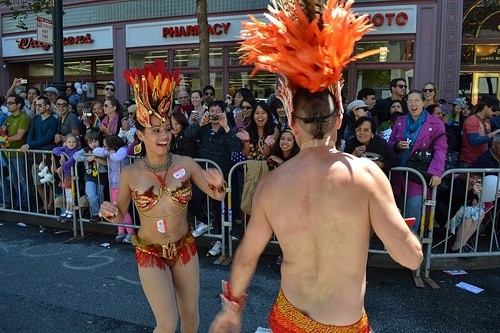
191;221;213;236
208;240;228;256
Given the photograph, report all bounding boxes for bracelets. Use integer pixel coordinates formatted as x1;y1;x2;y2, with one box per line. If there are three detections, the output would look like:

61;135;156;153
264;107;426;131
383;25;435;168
104;216;114;223
208;181;227;193
219;280;248;311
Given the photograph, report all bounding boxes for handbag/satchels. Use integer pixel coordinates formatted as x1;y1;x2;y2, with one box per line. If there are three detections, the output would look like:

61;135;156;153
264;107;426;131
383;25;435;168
403;132;456;193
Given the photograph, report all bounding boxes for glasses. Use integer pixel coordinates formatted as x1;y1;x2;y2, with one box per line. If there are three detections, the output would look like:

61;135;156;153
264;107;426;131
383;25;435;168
104;104;114;108
105;87;115;92
204;92;214;96
355;127;371;132
355;107;367;112
55;103;67;108
487;105;497;112
422;88;434;93
406;100;422;104
240;105;252;109
126;112;136;115
6;102;17;105
394;84;408;89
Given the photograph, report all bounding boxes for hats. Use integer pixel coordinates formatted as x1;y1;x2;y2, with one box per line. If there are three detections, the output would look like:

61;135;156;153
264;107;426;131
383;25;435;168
447;98;466;107
347;100;370;115
42;87;58;95
482;93;500;111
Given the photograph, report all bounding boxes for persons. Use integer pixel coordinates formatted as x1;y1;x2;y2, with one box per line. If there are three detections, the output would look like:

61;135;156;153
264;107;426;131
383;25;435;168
0;77;500;256
99;104;228;333
209;88;423;333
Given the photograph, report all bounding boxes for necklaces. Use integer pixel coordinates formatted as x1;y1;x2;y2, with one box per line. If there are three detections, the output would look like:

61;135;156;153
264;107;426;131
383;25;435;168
301;132;335;150
144;152;173;172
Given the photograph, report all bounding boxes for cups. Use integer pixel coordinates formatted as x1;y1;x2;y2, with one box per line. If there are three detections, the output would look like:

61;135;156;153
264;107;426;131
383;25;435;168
191;111;199;122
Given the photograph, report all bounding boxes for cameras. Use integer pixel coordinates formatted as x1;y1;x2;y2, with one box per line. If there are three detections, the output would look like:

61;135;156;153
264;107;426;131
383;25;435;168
209;114;218;120
21;79;27;83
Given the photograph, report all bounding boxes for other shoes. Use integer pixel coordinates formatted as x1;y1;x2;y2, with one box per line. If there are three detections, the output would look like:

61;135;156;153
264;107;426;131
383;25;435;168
90;215;100;222
116;231;136;244
36;207;51;215
56;211;74;223
